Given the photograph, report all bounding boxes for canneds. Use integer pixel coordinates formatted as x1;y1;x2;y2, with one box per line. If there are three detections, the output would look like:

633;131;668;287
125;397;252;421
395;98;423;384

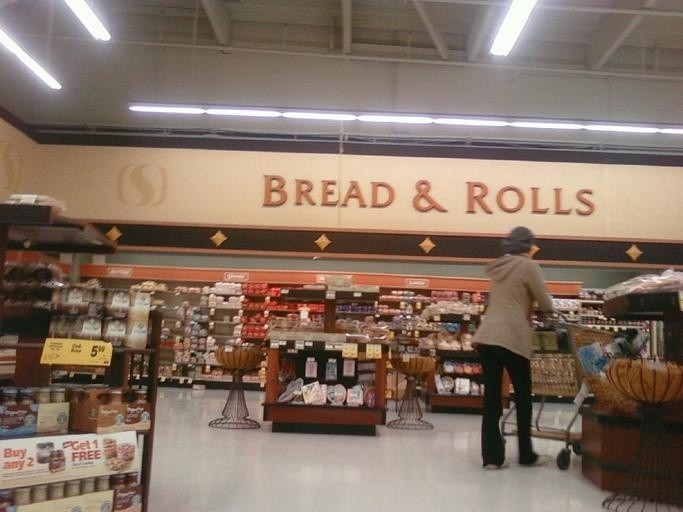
133;391;148;402
101;437;135;460
110;391;123;402
1;387;65;403
36;441;66;473
0;472;140;512
70;388;84;401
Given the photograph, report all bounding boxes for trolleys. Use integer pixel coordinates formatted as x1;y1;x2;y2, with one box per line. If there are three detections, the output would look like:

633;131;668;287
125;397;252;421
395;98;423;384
501;310;650;469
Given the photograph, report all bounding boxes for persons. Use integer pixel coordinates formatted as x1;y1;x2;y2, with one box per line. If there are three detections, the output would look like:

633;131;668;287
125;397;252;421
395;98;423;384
469;226;555;470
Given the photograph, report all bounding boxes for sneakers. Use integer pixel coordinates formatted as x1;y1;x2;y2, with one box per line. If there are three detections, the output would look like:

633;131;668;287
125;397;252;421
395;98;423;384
486;460;511;470
526;453;551;466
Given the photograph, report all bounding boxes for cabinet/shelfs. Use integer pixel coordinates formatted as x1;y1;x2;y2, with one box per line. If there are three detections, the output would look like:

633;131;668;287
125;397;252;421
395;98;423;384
2;207;152;392
265;321;387;433
327;272;507;412
54;260;330;384
505;283;583;398
0;311;159;512
581;299;655;363
